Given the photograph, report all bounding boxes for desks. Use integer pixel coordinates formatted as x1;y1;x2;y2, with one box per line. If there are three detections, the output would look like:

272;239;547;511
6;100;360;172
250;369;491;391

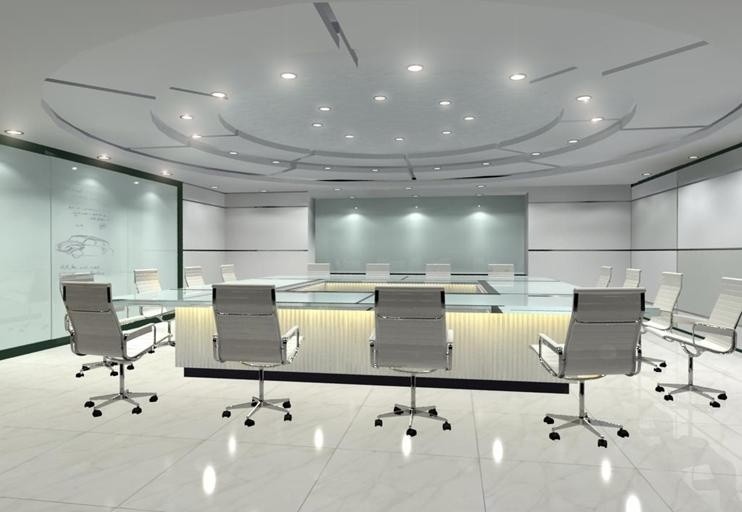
108;274;589;394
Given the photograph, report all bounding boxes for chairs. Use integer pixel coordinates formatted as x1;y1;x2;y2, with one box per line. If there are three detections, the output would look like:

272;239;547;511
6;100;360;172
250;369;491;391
644;275;741;408
630;271;684;374
209;281;304;427
365;284;453;440
596;265;614;287
218;264;238;280
486;263;516;277
132;269;176;355
60;280;171;418
365;264;391;276
621;267;643;287
183;266;206;287
424;264;452;277
528;284;649;449
305;264;332;275
59;272;136;378
